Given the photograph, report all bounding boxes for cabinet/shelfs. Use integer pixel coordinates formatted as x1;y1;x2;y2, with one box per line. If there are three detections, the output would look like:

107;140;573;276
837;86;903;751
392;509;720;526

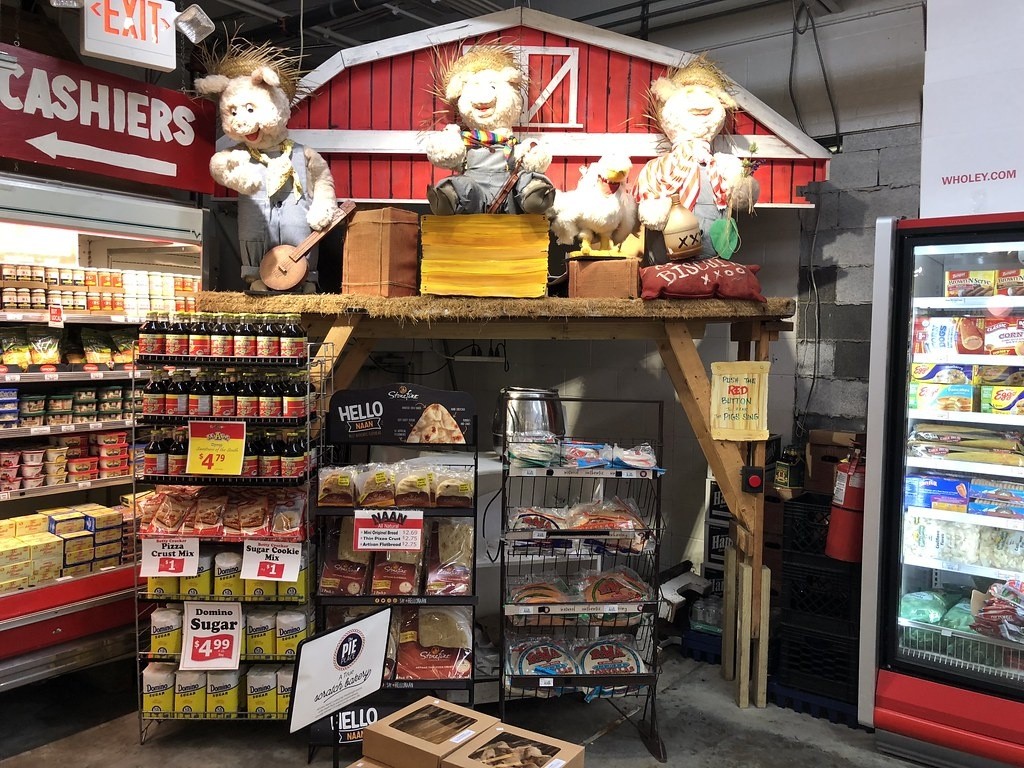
498;398;668;763
0;311;154;502
312;463;478;767
127;339;331;745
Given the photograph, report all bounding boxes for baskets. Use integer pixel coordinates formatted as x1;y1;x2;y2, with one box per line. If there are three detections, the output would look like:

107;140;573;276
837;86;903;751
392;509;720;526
780;489;861;705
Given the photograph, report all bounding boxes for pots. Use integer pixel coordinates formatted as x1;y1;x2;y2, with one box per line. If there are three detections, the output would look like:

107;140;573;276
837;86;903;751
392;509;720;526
491;386;566;459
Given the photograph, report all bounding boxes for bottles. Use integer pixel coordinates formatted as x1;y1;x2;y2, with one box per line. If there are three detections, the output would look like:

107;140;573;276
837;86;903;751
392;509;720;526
165;312;190;362
211;313;239;363
661;194;704;260
143;427;317;485
137;311;171;360
257;314;308;367
781;447;799;464
233;313;263;363
142;369;317;427
188;312;215;363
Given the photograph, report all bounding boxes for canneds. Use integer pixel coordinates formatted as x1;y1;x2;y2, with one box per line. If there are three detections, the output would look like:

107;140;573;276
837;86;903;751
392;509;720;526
0;264;201;313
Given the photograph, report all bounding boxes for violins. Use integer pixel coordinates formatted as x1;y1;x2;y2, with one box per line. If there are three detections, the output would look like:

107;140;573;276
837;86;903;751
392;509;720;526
486;141;537;214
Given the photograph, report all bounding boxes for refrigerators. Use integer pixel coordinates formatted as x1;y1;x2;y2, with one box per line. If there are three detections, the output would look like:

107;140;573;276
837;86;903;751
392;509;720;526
857;211;1024;768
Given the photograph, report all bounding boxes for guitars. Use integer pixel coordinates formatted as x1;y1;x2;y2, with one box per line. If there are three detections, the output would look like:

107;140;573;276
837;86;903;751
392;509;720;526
260;199;356;291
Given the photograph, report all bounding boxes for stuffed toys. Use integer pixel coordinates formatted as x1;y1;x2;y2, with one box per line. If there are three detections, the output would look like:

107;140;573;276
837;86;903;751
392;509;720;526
426;50;555;216
632;60;759;260
544;151;636;250
194;41;338;295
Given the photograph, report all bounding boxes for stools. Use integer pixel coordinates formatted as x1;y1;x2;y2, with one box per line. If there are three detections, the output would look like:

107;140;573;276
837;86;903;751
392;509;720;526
644;559;713;623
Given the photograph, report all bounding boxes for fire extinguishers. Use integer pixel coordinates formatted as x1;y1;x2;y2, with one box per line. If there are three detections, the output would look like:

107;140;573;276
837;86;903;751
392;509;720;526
823;438;864;565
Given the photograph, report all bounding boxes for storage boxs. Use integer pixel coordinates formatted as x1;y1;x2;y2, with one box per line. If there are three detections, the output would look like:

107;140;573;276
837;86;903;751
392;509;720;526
567;260;639;298
764;430;863;707
904;269;1023;521
347;695;586;768
342;207;419;298
420;213;549;299
1;490;153;596
699;465;733;600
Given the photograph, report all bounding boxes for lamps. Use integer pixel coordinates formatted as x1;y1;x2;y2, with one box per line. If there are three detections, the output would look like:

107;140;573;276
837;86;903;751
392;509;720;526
50;0;84;9
175;3;216;44
